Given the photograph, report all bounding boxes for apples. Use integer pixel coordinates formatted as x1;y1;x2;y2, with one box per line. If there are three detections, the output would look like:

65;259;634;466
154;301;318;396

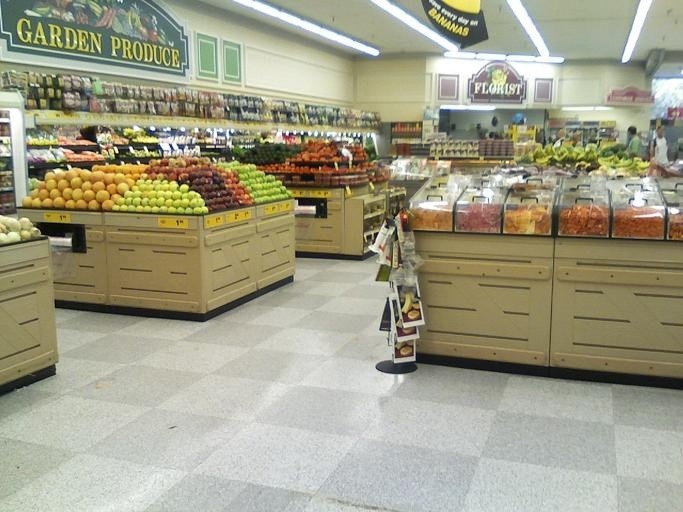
216;160;295;205
112;157;212;216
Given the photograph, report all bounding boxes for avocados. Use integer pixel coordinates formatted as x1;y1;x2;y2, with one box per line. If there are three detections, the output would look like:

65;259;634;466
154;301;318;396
239;143;304;165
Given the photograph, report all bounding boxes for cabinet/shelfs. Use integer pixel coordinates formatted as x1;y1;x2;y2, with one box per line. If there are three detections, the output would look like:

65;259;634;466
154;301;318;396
17;196;294;322
404;169;559;378
290;180;406;260
0;88;29;219
0;236;58;399
23;110;378;195
550;170;683;390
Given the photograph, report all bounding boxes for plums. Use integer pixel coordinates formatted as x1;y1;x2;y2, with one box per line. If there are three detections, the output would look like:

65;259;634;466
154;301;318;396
175;166;240;211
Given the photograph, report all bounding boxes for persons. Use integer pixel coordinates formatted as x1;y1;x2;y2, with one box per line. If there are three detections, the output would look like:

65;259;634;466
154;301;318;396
647;125;669;163
625;125;641;156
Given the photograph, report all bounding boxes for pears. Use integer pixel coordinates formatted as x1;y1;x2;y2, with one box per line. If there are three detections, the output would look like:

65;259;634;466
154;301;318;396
0;214;41;244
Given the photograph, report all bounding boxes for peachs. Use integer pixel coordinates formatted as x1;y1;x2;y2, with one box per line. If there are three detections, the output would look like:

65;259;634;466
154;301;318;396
256;140;367;174
